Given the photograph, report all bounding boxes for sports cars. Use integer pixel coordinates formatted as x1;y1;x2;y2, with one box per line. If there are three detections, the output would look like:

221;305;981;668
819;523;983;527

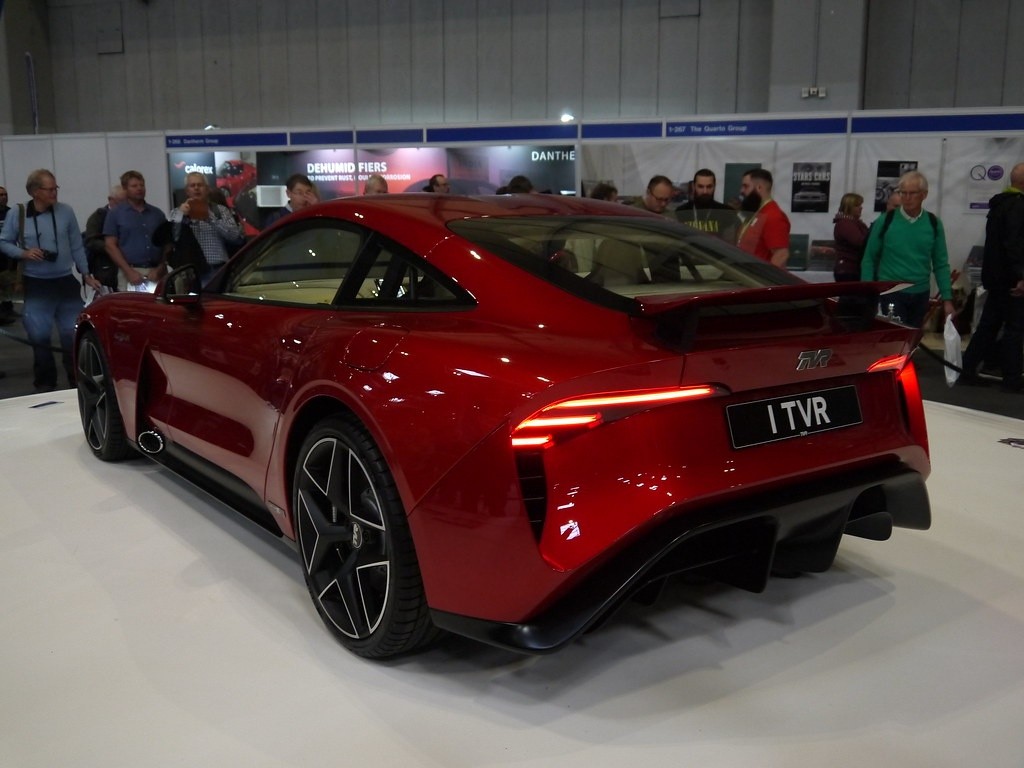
72;193;933;662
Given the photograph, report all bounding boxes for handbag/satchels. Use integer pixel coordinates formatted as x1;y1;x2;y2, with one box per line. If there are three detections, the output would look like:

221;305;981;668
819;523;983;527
0;270;21;303
832;244;857;278
942;314;964;389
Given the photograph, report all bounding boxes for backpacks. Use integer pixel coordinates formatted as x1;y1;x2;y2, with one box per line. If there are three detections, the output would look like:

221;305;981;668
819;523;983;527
75;232;89;273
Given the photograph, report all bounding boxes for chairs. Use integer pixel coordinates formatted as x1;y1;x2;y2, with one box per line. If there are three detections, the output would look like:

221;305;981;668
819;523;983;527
590;237;682;288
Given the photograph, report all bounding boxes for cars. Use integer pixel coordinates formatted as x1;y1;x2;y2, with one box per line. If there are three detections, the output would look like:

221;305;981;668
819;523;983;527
214;160;257;196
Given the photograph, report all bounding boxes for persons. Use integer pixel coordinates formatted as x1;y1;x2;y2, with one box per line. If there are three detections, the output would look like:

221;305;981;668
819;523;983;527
362;174;389;195
0;170;247;396
420;175;451;192
496;168;902;284
263;173;320;229
865;173;956;332
949;162;1024;389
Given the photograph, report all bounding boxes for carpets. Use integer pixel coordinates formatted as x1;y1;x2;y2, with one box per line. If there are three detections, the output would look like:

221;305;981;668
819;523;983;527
912;349;1024;422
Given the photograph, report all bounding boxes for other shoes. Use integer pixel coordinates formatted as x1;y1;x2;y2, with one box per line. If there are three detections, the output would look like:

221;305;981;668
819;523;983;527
956;369;993;387
0;316;15;325
0;301;19;316
999;377;1024;393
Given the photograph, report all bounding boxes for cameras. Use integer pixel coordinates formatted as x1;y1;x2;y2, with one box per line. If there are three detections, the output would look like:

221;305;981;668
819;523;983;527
40;248;58;262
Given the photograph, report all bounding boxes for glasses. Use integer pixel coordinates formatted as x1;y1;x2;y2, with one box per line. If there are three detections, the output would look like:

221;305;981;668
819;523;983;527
649;192;670;203
290;191;311;197
895;189;918;199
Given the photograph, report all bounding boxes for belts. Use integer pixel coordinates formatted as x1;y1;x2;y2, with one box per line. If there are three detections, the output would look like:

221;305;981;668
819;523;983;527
128;263;159;268
